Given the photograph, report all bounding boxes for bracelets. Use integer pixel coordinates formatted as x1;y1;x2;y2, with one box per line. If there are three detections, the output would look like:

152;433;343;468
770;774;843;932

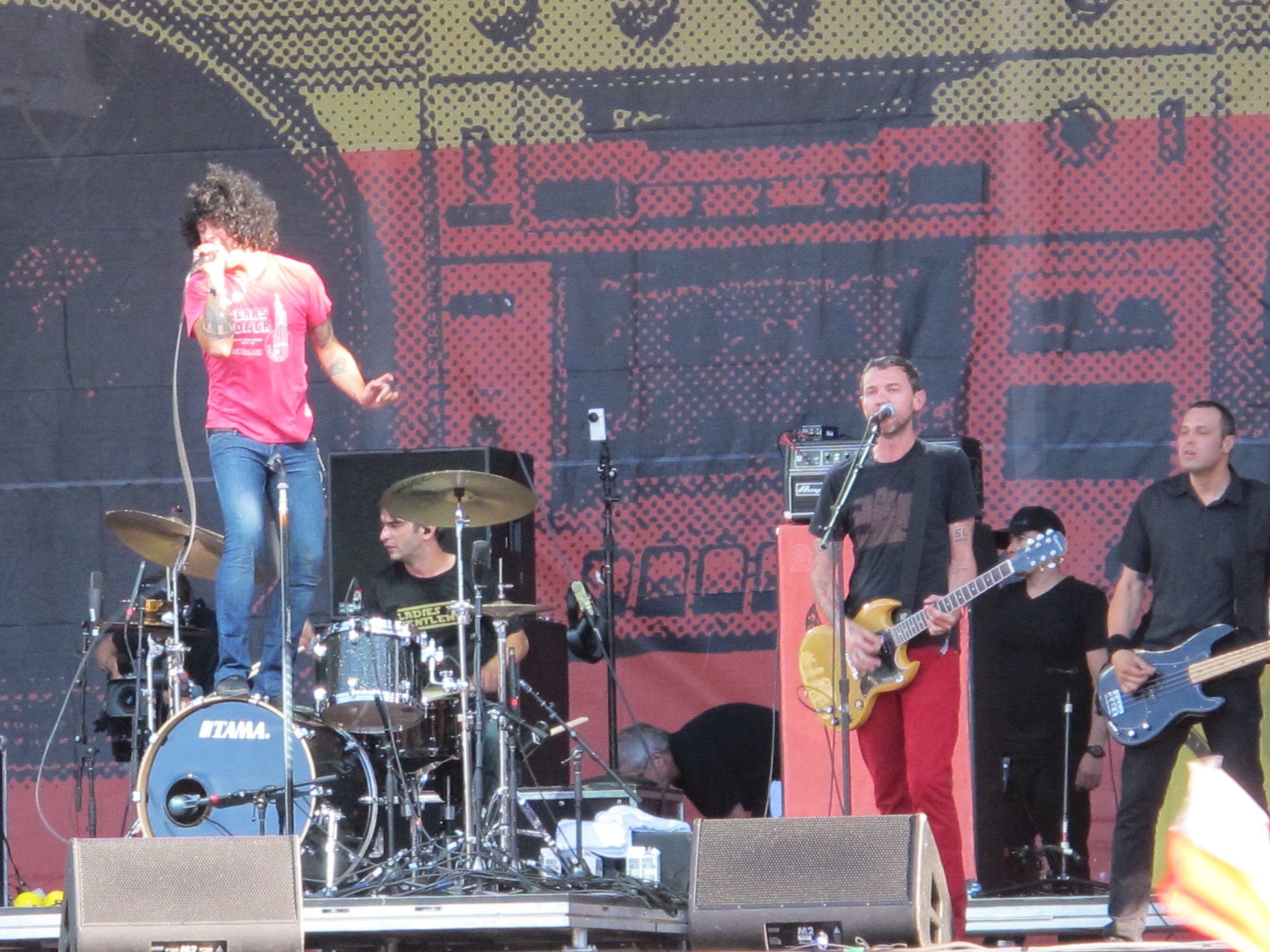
207;288;217;296
1108;634;1132;661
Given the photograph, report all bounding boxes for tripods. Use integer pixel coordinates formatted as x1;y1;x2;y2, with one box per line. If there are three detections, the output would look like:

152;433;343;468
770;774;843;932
468;552;575;891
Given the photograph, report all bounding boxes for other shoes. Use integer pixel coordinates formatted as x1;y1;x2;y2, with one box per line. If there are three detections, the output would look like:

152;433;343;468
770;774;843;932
217;675;249;699
270;690;316;717
1101;934;1129;942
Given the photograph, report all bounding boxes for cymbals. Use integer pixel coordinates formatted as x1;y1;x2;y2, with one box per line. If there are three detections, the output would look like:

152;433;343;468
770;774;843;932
468;596;556;620
101;609;210;643
380;469;536;530
106;510;263;585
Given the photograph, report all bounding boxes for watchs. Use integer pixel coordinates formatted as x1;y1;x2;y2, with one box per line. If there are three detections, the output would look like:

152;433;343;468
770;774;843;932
1086;745;1105;758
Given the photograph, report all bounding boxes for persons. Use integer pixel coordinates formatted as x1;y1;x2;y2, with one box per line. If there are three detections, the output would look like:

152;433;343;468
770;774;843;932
978;505;1110;947
1105;401;1270;941
618;701;781;819
309;497;530;804
178;165;400;705
808;356;981;946
96;569;220;712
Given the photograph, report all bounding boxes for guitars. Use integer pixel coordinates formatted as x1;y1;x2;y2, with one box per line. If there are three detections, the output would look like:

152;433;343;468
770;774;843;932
1096;622;1269;748
798;529;1069;731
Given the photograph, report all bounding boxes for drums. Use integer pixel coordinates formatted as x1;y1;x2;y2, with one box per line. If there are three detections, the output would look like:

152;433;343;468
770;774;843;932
355;698;459;759
308;615;422;733
133;694;378;894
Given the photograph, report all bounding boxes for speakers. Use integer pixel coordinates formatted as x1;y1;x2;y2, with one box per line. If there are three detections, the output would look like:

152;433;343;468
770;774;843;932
309;618;568;864
58;835;304;952
687;812;953;952
328;445;534;622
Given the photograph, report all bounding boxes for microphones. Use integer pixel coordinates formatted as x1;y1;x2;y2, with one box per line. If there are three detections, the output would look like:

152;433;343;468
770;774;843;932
187;253;215;275
87;569;104;636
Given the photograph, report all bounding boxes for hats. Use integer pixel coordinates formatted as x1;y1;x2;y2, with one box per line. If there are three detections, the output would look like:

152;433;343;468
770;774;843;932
993;505;1067;550
